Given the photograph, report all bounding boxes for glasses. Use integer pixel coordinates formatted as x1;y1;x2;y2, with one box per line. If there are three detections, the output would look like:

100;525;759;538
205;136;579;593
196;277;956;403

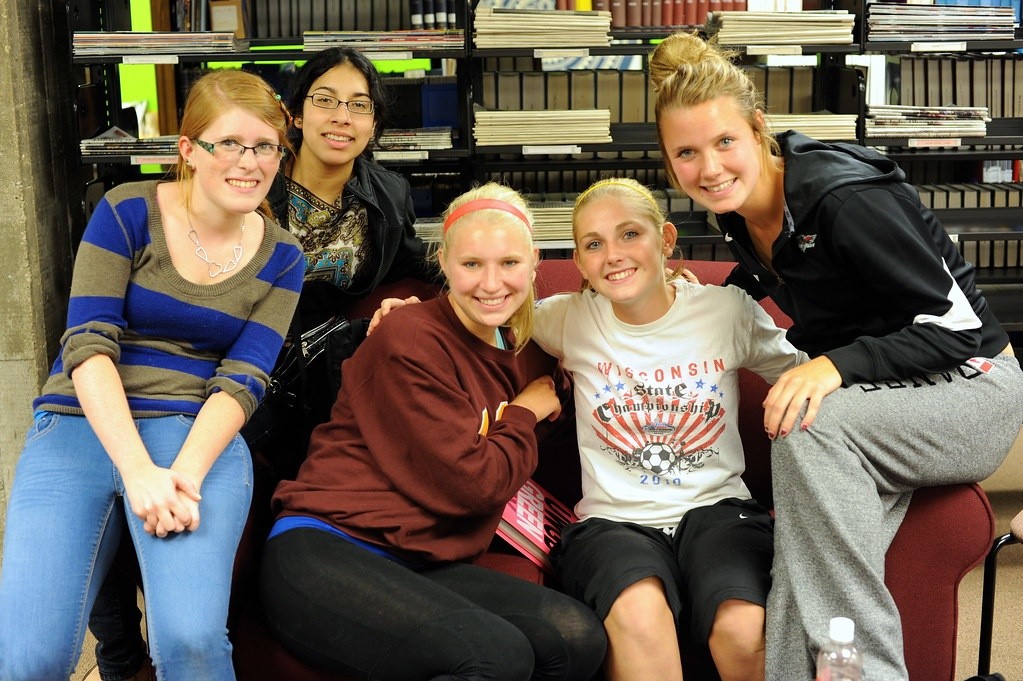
195;139;285;162
306;93;375;113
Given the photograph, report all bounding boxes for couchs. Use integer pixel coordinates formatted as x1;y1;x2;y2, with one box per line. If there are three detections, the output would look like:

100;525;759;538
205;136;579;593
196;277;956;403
347;251;999;681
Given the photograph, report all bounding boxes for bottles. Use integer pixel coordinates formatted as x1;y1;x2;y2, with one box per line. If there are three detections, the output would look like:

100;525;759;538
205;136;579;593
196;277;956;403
815;617;867;681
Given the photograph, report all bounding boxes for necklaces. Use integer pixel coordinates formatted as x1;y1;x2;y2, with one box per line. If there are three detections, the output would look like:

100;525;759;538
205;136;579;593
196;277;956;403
184;186;245;278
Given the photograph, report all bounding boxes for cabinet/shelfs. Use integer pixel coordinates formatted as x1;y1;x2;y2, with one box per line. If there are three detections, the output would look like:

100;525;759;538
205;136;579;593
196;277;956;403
22;0;1023;378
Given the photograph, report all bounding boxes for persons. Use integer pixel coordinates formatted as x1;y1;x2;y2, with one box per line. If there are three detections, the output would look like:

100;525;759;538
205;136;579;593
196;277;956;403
253;181;610;681
1;68;307;680
82;42;451;681
649;30;1021;681
363;177;812;681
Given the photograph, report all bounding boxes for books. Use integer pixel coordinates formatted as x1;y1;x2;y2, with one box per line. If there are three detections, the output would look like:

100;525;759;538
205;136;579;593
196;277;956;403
71;1;1021;271
492;476;579;571
